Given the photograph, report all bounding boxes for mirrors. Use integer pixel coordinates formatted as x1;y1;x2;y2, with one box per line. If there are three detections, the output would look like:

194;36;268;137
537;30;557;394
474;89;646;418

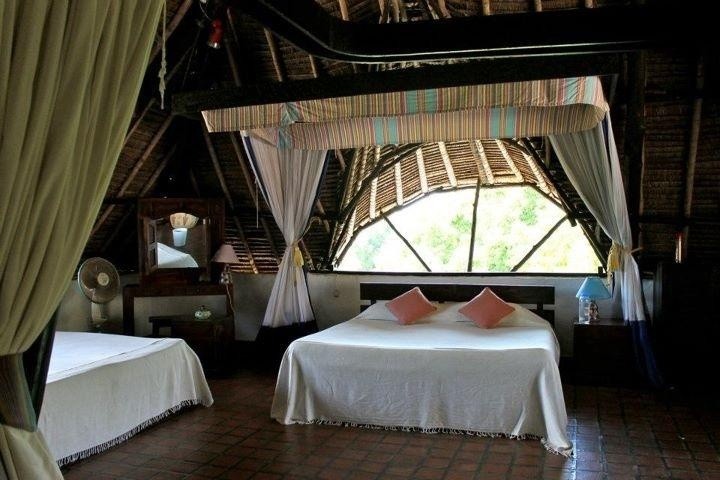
136;193;227;279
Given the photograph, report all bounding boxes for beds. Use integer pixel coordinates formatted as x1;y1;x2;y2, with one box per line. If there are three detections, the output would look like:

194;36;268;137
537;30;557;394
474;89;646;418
36;330;199;466
283;282;559;447
157;242;199;270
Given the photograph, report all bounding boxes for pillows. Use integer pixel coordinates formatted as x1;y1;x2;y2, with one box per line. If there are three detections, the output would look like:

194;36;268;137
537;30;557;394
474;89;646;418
383;286;436;325
457;288;518;328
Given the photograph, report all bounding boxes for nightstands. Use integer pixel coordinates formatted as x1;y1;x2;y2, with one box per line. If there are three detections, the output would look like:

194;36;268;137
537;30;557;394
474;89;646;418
569;317;638;392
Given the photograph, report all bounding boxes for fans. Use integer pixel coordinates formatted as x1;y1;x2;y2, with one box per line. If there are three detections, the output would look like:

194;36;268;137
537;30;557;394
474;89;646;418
79;258;121;331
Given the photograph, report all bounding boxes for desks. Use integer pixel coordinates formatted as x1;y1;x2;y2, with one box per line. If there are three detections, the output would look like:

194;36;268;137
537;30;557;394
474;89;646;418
148;315;235;380
122;280;235;341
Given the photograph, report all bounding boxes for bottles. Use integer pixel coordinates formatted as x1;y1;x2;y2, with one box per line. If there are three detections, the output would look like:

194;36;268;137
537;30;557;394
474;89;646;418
578;294;591;325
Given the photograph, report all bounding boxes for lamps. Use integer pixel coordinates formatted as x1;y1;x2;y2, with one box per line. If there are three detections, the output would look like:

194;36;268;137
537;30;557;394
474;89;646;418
205;19;221;49
575;276;611;322
212;244;240;320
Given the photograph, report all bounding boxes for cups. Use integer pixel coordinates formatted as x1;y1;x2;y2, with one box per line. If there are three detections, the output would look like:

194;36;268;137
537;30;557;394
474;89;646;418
173;228;187;246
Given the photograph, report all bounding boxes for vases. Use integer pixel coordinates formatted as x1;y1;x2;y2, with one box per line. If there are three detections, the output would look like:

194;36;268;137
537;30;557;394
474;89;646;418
195;310;212;320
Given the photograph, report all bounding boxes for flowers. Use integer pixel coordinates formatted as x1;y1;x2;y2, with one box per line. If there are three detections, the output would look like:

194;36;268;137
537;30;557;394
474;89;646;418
192;303;212;311
